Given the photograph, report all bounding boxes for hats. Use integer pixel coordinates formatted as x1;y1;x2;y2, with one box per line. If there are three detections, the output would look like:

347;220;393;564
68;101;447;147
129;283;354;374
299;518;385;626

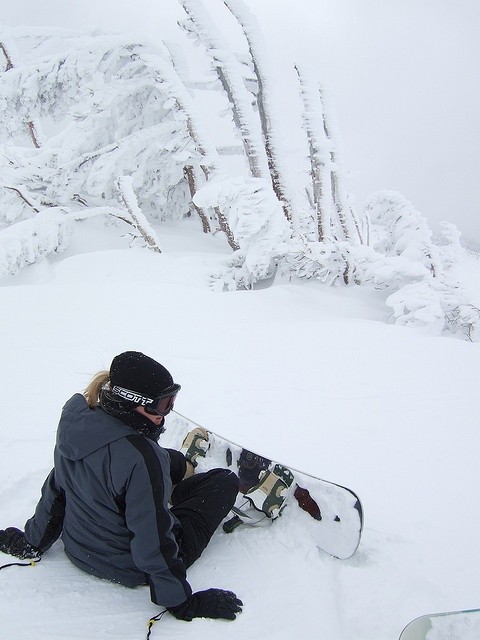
108;350;182;408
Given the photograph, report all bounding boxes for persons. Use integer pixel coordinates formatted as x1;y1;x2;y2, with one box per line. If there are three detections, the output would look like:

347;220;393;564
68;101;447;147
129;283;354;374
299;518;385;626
0;351;242;622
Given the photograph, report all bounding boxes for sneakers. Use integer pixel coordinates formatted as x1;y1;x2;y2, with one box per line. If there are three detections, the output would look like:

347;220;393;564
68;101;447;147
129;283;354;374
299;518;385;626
231;463;296;526
179;427;208;477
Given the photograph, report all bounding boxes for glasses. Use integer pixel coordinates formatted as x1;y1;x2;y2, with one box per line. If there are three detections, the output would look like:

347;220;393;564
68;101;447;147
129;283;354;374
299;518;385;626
108;395;177;416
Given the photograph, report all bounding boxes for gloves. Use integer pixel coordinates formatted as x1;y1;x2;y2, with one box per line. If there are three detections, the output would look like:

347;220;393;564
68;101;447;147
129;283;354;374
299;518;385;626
165;588;243;621
1;527;43;559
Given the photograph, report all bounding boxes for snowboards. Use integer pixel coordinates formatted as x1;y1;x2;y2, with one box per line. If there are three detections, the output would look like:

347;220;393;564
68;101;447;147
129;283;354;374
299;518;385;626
399;609;480;640
157;409;363;560
